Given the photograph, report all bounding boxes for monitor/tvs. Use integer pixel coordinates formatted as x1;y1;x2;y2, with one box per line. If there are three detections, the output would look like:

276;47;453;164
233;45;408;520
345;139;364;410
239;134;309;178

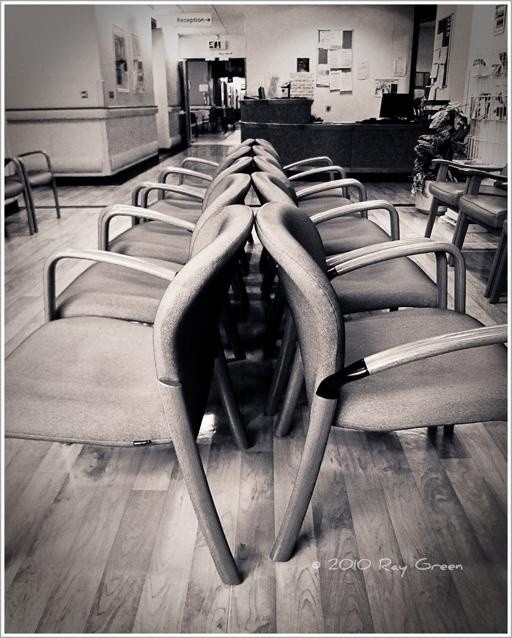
380;94;413;124
258;87;265;99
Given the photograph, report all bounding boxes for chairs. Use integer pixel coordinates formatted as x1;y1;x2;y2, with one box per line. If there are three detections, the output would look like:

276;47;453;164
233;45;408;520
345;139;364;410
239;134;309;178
5;135;508;585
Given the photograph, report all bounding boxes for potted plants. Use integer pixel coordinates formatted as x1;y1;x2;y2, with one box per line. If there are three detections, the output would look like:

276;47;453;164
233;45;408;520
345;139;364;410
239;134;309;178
411;101;469;216
